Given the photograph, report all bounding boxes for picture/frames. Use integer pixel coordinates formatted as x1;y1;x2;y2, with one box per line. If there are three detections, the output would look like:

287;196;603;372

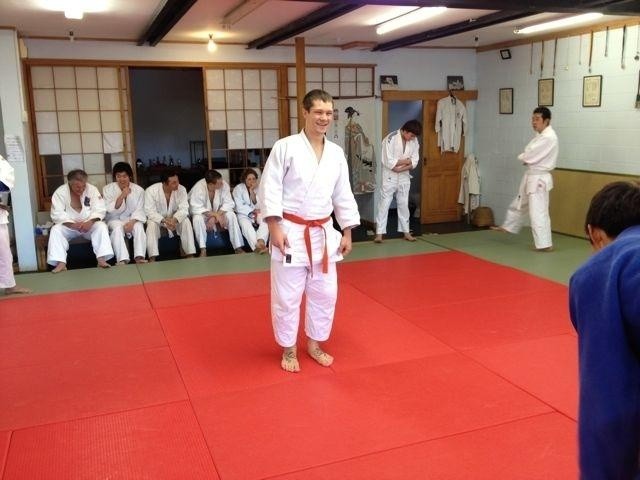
498;88;513;115
538;78;554;107
583;75;602;108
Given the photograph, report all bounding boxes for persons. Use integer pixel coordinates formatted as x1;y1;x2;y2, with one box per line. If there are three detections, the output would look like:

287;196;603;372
102;161;150;266
566;179;640;479
47;168;114;275
0;154;36;296
489;105;560;253
142;168;199;265
231;170;270;254
372;119;422;244
258;89;362;374
186;170;247;257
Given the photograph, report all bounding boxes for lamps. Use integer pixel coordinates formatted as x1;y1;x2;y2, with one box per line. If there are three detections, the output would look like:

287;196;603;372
374;5;449;36
513;8;604;36
207;32;217;52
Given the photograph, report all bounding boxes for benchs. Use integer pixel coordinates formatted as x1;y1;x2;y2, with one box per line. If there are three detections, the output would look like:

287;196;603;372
35;218;271;273
